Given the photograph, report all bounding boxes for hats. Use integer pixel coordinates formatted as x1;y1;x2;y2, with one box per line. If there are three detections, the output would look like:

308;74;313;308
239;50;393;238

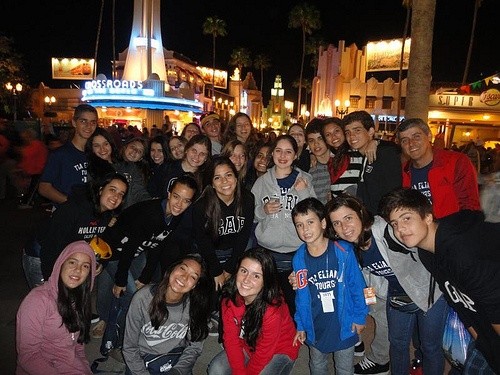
200;111;220;125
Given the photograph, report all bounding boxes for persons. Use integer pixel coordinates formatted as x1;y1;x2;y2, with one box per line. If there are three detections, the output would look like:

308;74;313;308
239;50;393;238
442;140;500;188
397;119;481;222
382;188;500;375
207;248;301;375
342;113;404;374
122;253;208;375
0;104;398;362
288;194;452;374
13;240;96;375
292;197;371;375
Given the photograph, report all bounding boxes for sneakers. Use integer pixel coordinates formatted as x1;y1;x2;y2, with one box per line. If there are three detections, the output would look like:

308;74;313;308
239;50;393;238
354;342;365;357
92;320;106;339
354;358;390;375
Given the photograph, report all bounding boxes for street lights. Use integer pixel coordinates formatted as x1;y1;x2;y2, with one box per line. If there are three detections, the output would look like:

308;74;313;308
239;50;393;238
335;99;350;120
6;82;22;122
44;96;56;123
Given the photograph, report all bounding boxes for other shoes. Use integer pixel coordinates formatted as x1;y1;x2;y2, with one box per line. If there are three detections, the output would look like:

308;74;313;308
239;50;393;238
100;340;116;356
207;318;220;337
91;313;100;323
